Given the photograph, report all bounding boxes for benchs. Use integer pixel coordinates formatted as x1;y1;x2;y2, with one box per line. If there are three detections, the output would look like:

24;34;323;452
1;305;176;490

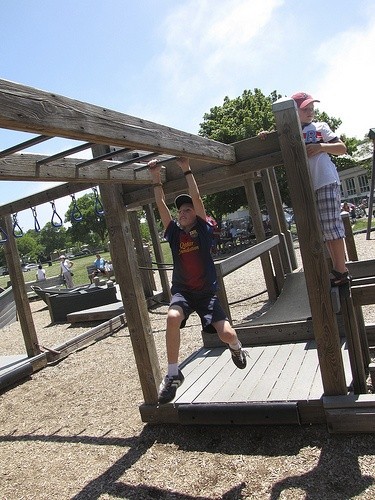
24;273;67;301
86;261;115;284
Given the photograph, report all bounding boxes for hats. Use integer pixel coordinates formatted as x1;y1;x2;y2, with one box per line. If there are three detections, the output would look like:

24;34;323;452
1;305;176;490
59;255;66;259
174;194;193;210
291;92;320;108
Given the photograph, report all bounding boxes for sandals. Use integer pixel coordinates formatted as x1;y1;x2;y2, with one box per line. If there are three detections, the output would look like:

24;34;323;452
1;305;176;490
329;269;350;287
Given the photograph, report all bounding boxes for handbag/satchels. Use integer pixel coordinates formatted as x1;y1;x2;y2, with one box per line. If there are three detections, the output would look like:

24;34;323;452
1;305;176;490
70;272;73;276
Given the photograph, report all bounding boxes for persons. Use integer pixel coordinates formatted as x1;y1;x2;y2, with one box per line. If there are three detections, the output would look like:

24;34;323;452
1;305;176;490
141;238;153;259
58;255;74;289
93;254;110;280
340;198;375;224
257;92;354;288
211;209;296;254
146;156;247;404
36;265;46;282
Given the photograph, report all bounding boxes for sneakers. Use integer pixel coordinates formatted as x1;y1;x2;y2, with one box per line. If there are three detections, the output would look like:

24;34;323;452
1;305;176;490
158;368;184;404
226;339;252;369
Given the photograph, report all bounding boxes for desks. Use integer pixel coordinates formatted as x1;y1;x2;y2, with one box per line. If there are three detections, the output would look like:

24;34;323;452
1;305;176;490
216;227;274;255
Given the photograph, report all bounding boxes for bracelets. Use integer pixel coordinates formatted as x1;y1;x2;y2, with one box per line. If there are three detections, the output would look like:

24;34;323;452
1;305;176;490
152;183;163;187
183;170;192;176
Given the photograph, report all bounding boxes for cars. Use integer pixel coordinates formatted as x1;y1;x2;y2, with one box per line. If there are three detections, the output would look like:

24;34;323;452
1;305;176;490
0;266;9;276
22;263;39;272
67;251;86;258
